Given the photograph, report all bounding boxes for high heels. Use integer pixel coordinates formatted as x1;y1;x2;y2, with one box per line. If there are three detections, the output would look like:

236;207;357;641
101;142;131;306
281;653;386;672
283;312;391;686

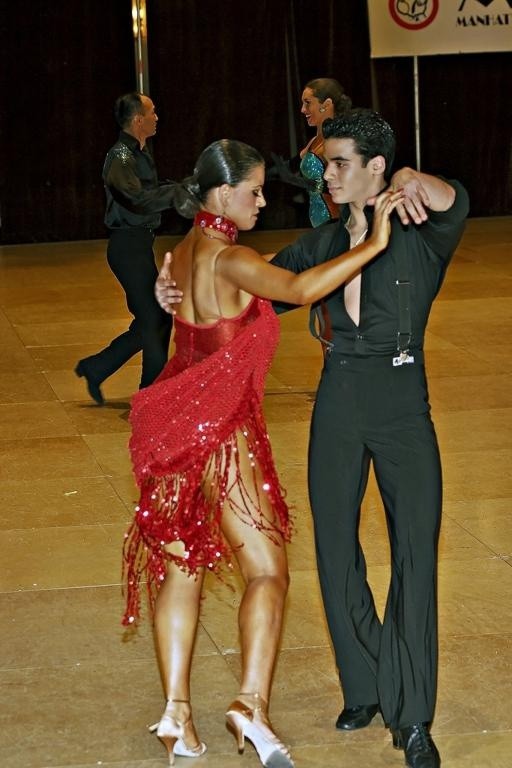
225;691;296;768
148;699;208;766
75;359;104;404
392;723;441;768
335;705;380;730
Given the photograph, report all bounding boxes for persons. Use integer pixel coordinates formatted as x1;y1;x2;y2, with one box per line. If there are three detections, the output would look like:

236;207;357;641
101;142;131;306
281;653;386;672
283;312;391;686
263;77;352;229
74;91;188;406
121;138;407;768
154;109;469;768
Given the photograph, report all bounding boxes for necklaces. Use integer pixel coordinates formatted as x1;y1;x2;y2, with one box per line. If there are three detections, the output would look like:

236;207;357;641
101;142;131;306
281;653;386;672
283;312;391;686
193;208;240;243
355;228;368;247
